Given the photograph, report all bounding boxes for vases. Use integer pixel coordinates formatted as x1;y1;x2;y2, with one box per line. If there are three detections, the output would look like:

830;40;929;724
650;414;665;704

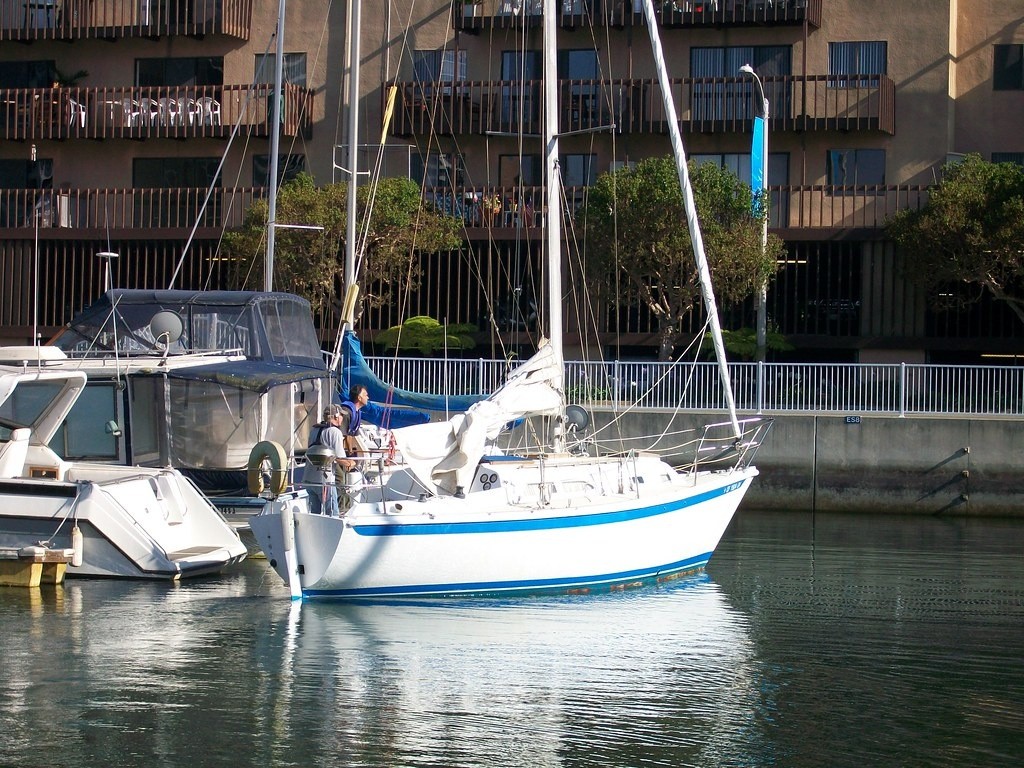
477;208;494;228
509;204;517;212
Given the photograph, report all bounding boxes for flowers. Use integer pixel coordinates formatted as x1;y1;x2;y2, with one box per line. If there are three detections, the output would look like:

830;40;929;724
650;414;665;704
508;198;517;205
474;193;502;215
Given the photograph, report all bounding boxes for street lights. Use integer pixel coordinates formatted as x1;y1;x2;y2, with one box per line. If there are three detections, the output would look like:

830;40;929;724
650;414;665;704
738;62;771;411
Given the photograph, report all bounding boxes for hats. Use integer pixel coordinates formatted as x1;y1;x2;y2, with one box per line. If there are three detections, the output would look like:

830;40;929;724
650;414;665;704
324;404;348;416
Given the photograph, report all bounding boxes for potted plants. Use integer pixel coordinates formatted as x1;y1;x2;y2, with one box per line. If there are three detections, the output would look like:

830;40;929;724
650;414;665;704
456;0;485;17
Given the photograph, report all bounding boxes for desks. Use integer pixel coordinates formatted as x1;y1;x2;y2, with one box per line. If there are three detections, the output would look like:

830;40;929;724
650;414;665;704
22;3;58;29
505;211;536;227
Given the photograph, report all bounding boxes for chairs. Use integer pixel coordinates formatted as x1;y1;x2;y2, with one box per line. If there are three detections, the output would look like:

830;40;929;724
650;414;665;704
160;98;183;127
196;97;221;126
120;98;143;127
178;97;203;126
138;98;163;127
70;99;86;128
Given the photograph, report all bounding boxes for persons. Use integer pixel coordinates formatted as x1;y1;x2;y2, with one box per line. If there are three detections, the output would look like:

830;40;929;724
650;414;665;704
338;385;369;518
304;403;355;519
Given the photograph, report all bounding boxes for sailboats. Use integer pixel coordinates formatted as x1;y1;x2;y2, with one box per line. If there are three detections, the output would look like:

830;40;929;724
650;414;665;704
0;0;773;598
